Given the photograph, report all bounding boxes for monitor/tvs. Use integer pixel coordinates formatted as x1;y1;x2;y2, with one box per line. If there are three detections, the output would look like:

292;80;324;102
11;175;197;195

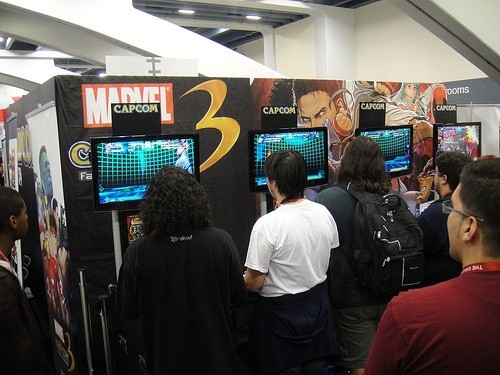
90;133;201;212
247;127;329;193
433;122;482;169
355;124;413;178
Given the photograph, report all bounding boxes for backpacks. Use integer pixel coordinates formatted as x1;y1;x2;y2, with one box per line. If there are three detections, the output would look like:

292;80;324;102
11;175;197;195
336;180;426;302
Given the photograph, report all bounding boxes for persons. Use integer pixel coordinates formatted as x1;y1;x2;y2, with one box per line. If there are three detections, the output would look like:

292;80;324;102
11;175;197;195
0;186;53;375
417;151;474;286
115;166;248;375
315;135;409;375
243;150;340;375
363;158;500;375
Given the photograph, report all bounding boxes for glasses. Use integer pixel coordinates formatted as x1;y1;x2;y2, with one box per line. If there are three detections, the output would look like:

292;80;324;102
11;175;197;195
431;170;437;173
442;199;484;224
